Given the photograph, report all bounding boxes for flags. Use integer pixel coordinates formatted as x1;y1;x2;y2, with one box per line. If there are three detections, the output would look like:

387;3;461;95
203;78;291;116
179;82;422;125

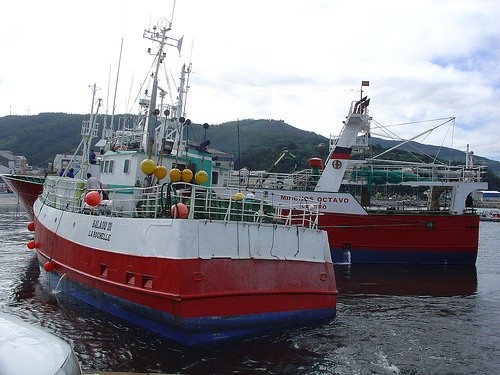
362;81;370;86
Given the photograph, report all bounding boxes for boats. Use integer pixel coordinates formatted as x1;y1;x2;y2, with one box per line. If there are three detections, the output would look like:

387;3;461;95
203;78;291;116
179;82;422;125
28;0;339;347
0;82;488;270
0;152;47;194
367;193;499;223
0;313;81;375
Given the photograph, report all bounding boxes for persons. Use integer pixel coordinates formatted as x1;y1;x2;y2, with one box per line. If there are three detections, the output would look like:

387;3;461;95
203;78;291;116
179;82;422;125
59;169;65;177
67;168;74;178
89;150;96;163
86;173;110;208
246;192;255;198
